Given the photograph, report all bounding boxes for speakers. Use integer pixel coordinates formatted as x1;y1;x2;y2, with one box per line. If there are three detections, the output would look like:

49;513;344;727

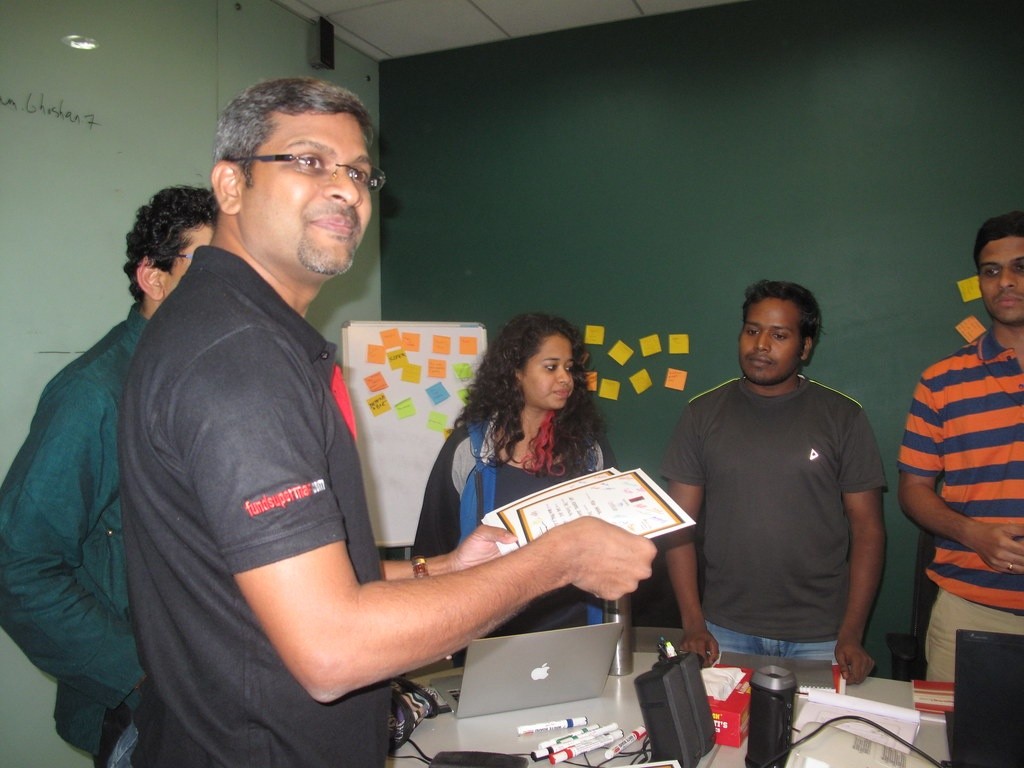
633;653;716;768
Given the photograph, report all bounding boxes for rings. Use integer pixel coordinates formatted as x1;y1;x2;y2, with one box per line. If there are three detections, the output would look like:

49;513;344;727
1007;564;1013;570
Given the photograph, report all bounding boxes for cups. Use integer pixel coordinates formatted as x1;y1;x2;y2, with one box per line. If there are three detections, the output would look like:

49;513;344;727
653;650;704;670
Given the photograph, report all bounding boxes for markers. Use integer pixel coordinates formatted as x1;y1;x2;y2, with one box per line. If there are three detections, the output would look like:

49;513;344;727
656;636;678;660
516;715;588;735
529;723;647;763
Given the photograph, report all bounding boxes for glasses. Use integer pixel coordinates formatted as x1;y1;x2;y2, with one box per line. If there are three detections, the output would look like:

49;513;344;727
225;153;387;192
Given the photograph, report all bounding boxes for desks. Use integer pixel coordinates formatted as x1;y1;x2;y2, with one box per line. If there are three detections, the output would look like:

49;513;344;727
386;628;950;768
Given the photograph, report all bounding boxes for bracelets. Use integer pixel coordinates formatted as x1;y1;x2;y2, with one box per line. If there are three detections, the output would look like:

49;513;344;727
411;555;431;580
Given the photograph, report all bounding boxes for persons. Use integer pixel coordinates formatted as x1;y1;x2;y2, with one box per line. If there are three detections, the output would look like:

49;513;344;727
113;76;657;768
2;181;233;768
409;314;615;669
897;211;1024;682
659;279;890;684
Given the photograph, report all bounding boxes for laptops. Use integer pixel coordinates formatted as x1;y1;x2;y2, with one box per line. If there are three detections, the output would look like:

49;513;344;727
430;621;623;718
945;627;1024;768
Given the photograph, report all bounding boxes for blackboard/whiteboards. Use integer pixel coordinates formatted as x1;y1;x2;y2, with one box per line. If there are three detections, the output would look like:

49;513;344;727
341;318;489;549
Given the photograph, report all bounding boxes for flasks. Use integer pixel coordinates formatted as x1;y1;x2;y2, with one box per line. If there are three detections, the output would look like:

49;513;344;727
602;593;634;674
